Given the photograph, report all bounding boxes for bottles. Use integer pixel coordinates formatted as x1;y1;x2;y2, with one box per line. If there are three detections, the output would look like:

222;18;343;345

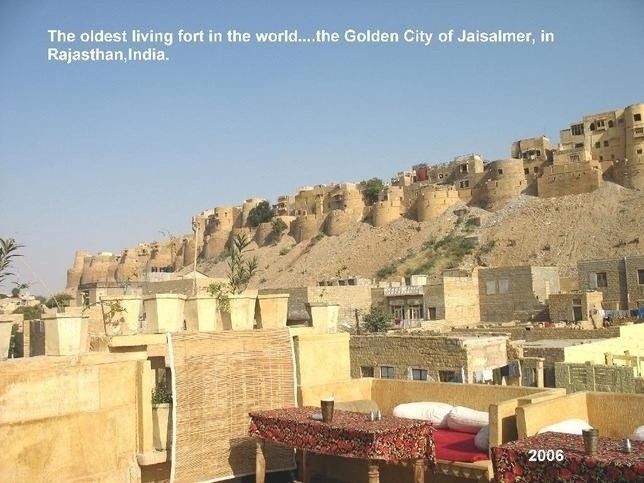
623;438;632;454
370;410;382;421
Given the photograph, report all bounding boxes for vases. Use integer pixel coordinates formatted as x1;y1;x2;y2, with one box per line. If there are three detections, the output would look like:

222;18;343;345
308;263;348;335
255;291;290;329
100;293;218;336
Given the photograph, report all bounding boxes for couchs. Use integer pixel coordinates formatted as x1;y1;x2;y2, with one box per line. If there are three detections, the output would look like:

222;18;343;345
295;376;644;483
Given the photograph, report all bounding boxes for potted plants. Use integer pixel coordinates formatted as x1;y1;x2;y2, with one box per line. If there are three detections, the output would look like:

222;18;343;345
150;379;171;451
214;237;258;330
0;238;126;355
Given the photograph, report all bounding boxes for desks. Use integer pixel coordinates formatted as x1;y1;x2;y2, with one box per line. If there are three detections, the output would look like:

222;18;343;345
247;406;436;483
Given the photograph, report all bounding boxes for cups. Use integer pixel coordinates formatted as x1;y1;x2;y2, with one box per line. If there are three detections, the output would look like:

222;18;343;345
321;401;334;423
582;428;599;456
